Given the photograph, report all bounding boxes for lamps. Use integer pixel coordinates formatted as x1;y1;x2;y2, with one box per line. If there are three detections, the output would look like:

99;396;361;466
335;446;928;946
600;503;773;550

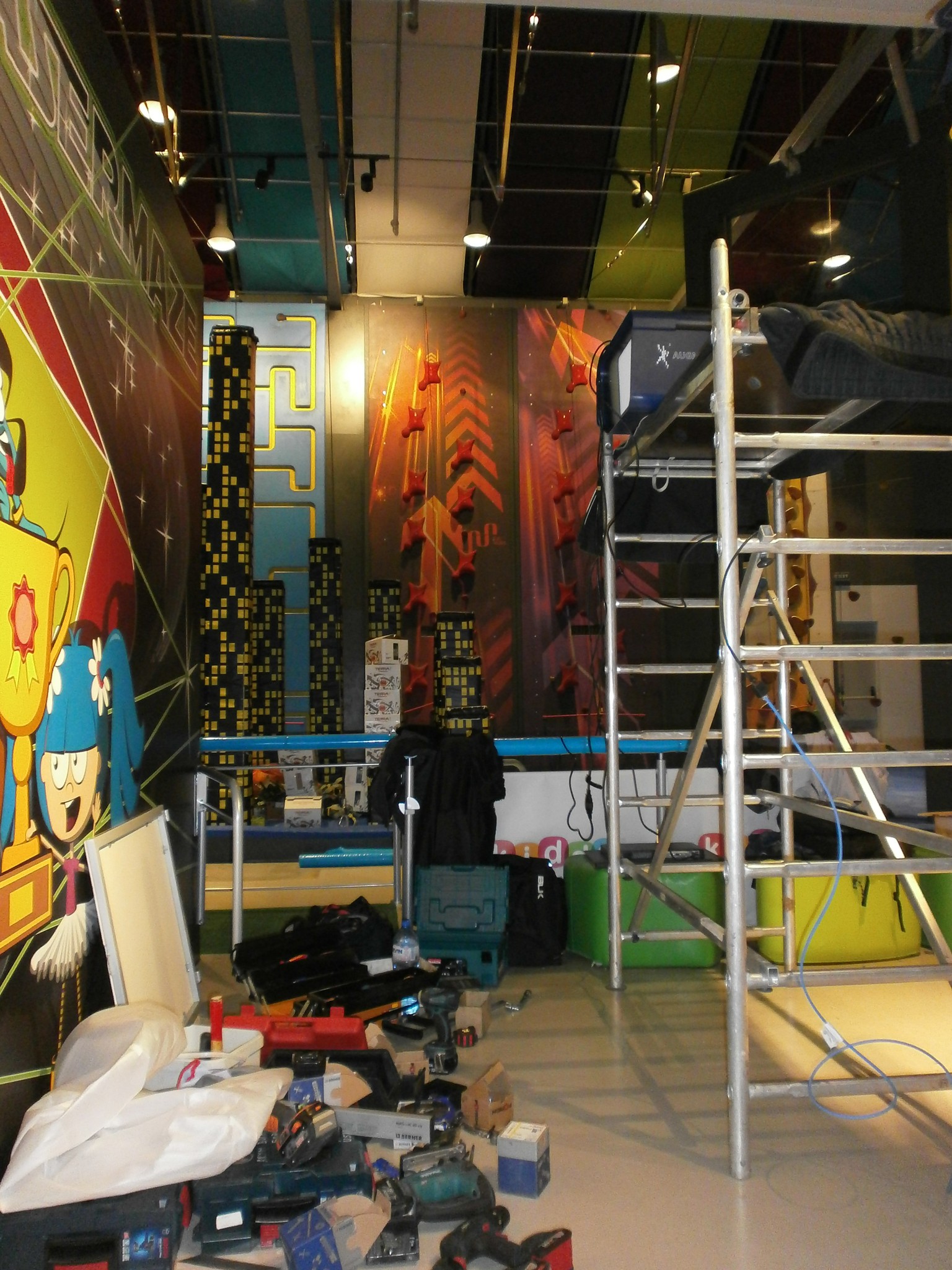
138;46;177;126
629;174;653;208
253;158;276;190
463;190;492;250
819;182;850;268
207;185;236;253
646;8;680;84
361;160;376;193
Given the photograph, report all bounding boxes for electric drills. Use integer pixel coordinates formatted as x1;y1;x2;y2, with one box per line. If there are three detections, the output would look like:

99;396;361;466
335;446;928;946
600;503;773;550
417;987;462;1075
440;1205;531;1270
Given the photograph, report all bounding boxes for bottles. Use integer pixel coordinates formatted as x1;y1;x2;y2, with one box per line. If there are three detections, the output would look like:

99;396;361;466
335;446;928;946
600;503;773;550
392;918;419;970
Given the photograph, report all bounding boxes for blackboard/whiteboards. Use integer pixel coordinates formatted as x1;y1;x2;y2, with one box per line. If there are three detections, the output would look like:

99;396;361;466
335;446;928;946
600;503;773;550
84;805;203;1026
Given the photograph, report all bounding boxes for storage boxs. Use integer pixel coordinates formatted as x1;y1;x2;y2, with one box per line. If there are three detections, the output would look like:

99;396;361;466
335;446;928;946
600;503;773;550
410;859;512;985
565;849;923;970
